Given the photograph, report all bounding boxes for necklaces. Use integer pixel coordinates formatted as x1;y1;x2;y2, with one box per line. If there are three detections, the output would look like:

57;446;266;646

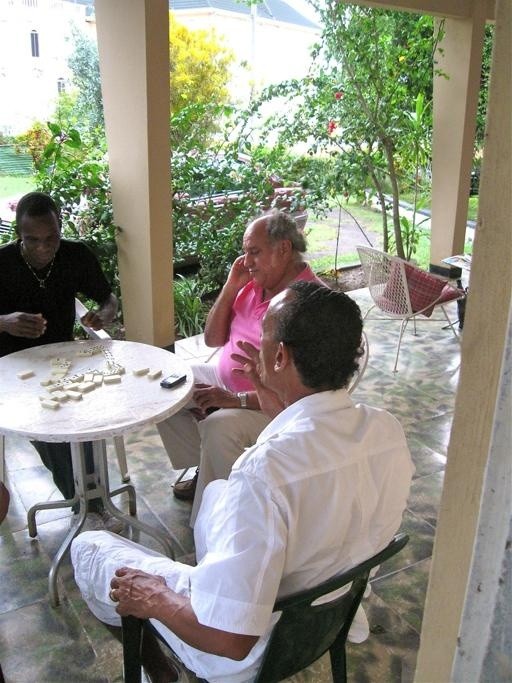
19;240;57;290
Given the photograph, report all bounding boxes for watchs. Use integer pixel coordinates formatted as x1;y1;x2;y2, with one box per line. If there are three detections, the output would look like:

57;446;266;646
236;389;249;408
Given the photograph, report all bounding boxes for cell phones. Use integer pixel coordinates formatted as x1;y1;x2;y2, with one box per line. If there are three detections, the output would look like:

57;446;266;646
159;371;188;388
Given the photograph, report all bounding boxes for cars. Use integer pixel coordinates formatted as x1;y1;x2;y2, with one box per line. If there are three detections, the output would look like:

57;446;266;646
409;153;479;195
0;146;309;279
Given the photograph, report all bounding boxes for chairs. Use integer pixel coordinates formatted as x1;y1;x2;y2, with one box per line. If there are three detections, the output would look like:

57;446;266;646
0;296;131;481
122;532;410;683
354;243;465;374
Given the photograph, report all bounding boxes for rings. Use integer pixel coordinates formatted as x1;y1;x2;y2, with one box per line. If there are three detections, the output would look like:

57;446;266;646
109;588;118;602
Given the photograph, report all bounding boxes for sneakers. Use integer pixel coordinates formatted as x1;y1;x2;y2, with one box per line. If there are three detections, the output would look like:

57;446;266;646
172;469;199;501
142;656;191;683
71;507;126;534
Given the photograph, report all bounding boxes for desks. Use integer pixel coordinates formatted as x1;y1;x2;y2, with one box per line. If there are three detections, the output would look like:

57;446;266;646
1;338;194;608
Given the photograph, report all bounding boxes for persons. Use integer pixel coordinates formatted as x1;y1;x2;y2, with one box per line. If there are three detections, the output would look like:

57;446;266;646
0;190;122;536
66;278;419;683
155;208;334;530
